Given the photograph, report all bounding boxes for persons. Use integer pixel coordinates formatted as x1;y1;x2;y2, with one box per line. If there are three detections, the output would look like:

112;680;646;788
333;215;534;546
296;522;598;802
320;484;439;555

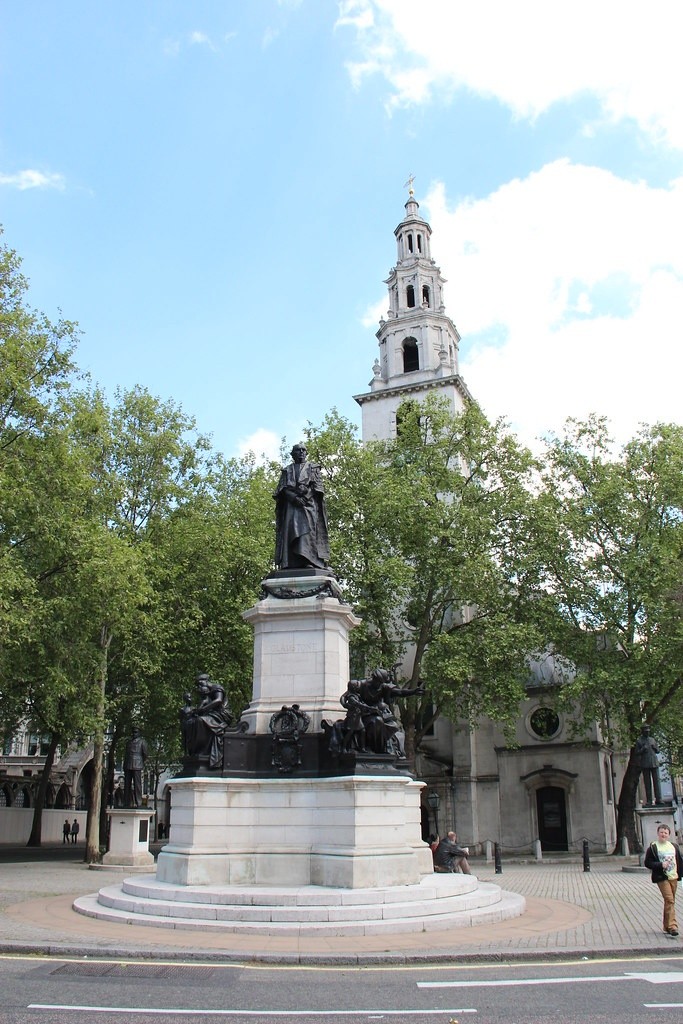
114;776;131;808
63;820;71;844
177;692;195;750
188;674;231;756
644;824;683;936
158;820;164;839
273;443;331;569
124;726;151;806
425;833;439;853
635;723;665;805
340;669;430;755
71;819;79;843
432;832;472;875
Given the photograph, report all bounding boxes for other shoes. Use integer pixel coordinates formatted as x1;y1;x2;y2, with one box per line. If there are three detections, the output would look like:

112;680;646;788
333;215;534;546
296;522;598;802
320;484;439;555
670;929;679;936
